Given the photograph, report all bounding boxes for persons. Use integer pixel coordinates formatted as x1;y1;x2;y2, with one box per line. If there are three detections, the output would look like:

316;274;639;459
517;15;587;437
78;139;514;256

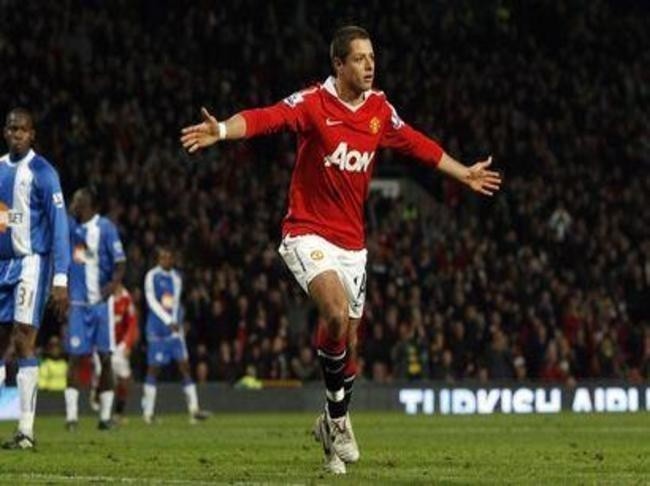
179;24;501;476
64;185;127;431
2;0;649;392
141;244;214;426
111;280;140;414
1;110;70;450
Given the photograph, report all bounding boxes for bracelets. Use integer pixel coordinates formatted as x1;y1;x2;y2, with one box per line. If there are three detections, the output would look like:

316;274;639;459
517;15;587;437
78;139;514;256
215;118;230;137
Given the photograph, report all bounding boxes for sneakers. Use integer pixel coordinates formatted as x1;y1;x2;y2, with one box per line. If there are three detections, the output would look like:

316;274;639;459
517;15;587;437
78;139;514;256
97;420;120;430
2;431;35;450
311;416;346;476
324;402;360;462
189;410;212;424
87;383;100;412
65;421;78;433
